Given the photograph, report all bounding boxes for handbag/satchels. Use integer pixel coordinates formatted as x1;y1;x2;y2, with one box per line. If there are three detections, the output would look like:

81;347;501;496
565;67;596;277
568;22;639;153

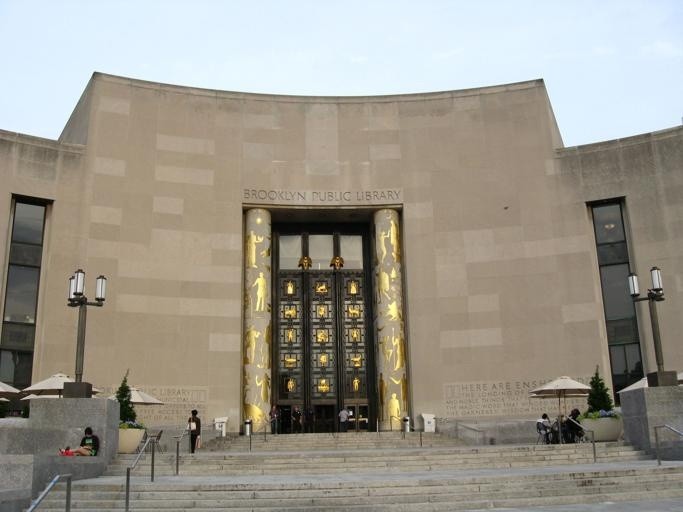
196;435;203;450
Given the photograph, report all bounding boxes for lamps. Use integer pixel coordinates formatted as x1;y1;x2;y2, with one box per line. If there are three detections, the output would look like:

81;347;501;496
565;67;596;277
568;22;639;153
328;257;345;270
296;255;312;271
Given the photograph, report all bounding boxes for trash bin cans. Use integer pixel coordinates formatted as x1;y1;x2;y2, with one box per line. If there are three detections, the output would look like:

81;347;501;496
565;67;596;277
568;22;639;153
244;420;252;436
403;417;410;432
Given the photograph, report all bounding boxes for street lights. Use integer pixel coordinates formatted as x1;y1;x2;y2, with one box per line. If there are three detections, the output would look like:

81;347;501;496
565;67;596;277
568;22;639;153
63;268;107;399
627;265;678;387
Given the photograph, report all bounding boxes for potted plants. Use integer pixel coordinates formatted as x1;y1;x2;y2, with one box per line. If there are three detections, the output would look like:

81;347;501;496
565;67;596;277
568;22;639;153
582;363;623;441
115;367;145;454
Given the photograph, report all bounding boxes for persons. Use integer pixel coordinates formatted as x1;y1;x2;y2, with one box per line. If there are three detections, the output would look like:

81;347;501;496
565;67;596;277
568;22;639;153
292;405;302;433
306;407;315;432
338;408;349;431
537;408;583;444
59;427;100;456
188;410;201;453
270;405;278;433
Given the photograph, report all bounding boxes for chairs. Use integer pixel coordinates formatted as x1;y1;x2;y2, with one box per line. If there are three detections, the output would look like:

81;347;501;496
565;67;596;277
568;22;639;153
133;429;164;454
535;420;584;445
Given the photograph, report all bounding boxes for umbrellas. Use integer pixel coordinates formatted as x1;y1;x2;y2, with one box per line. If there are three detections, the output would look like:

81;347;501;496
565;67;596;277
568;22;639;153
617;375;683;395
22;373;101;394
528;394;589;416
107;385;164;406
0;382;20;398
529;376;592;442
0;395;13;404
20;393;98;401
